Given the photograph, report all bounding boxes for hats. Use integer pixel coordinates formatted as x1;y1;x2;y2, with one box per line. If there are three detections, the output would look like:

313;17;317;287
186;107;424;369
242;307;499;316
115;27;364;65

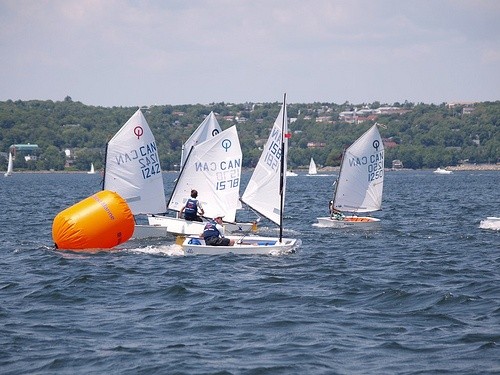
212;214;225;220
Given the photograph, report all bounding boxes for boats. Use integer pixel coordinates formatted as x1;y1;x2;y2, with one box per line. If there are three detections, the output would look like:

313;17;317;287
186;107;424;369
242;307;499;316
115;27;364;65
478;216;500;234
433;166;453;177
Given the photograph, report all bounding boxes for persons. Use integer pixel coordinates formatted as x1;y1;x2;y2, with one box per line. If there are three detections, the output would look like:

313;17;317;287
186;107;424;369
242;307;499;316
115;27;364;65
179;190;204;223
329;200;341;218
200;214;234;245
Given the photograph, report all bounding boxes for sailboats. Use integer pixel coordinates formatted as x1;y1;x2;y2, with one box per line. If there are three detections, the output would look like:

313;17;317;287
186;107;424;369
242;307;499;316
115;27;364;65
305;156;322;176
183;93;297;252
99;109;167;239
3;151;15;178
147;111;255;233
306;122;385;227
86;161;98;178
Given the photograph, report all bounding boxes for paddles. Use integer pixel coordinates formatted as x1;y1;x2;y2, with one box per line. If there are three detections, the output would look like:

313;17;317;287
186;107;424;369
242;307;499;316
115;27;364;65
236;217;261;244
197;212;233;234
